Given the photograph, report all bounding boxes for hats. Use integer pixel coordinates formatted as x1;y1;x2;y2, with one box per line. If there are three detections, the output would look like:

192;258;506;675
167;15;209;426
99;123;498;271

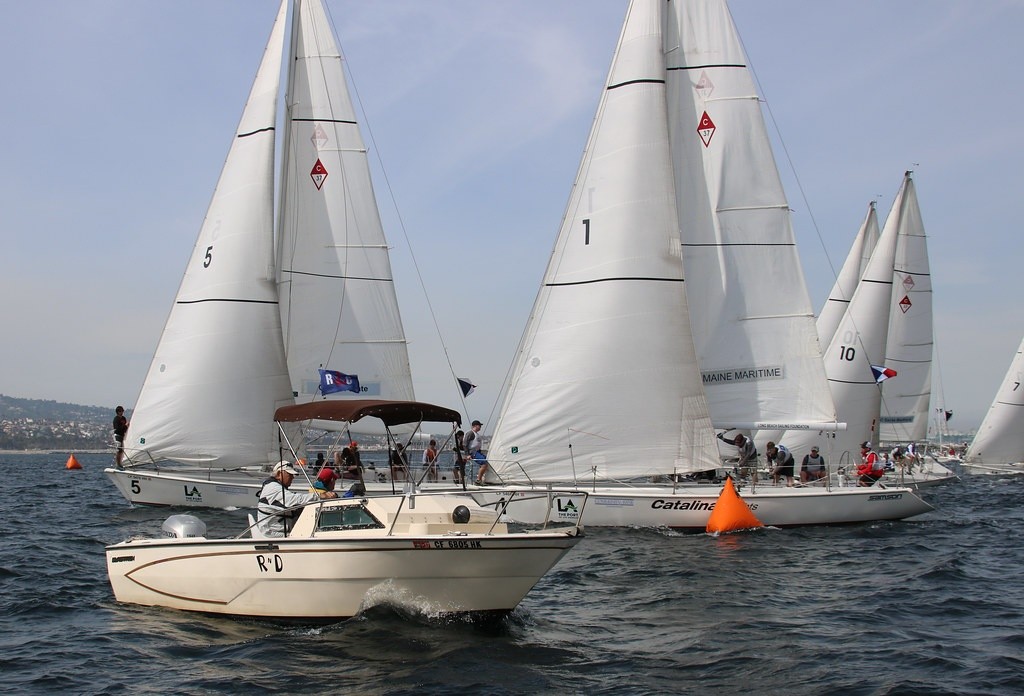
472;420;483;426
350;442;357;446
273;461;299;476
860;441;872;448
811;446;819;452
319;469;338;480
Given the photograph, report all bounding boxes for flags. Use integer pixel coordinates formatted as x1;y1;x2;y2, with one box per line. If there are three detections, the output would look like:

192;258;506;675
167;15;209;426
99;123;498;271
457;377;478;398
318;369;361;396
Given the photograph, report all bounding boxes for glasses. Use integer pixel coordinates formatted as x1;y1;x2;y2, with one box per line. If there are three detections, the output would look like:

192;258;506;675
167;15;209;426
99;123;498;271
118;410;124;412
276;462;293;470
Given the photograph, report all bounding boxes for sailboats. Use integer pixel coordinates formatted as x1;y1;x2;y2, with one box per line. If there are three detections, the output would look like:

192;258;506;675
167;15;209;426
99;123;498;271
814;172;1023;486
464;0;934;528
100;1;477;511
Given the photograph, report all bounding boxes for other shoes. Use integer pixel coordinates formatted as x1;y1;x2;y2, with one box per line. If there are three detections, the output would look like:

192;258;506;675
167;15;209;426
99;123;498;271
116;465;125;471
475;479;489;486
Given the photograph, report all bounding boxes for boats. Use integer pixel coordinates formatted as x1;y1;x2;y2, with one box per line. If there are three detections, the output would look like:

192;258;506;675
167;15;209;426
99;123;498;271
105;397;587;631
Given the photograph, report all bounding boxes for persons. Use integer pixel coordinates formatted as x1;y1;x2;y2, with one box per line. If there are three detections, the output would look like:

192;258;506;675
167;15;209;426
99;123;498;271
309;441;366;497
716;430;758;495
422;439;440;483
452;420;489;486
801;446;826;483
876;441;915;472
949;448;955;455
257;460;338;538
388;443;411;480
766;441;795;487
852;441;883;487
112;406;129;471
